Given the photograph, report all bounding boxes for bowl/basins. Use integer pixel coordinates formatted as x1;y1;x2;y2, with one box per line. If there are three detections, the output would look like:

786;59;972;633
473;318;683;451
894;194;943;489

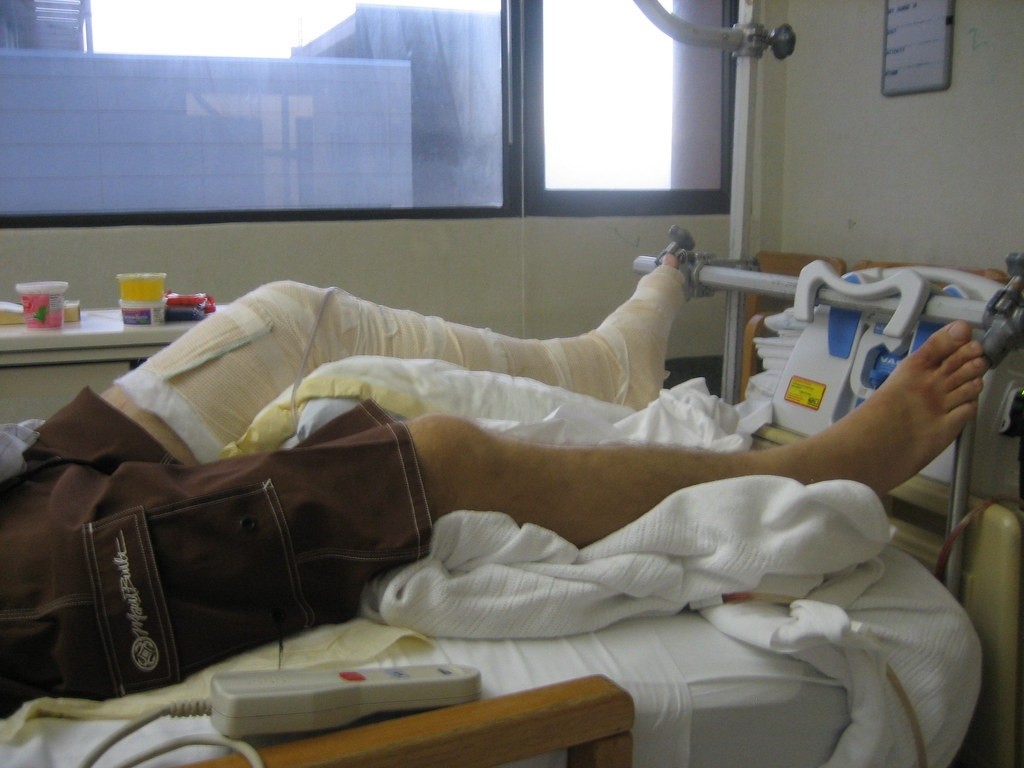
115;273;167;303
117;299;168;326
14;281;70;329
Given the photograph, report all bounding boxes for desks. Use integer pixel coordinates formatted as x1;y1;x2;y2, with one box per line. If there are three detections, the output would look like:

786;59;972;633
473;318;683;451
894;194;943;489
0;300;229;428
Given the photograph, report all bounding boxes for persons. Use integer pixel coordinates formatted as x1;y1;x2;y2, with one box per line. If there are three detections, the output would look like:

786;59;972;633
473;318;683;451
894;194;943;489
0;250;989;721
